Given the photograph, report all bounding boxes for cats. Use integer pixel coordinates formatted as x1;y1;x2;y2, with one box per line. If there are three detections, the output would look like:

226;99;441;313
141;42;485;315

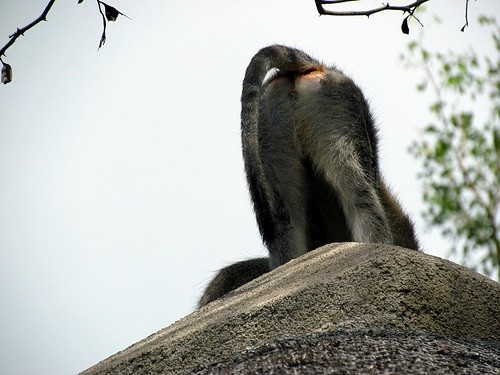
198;45;420;307
